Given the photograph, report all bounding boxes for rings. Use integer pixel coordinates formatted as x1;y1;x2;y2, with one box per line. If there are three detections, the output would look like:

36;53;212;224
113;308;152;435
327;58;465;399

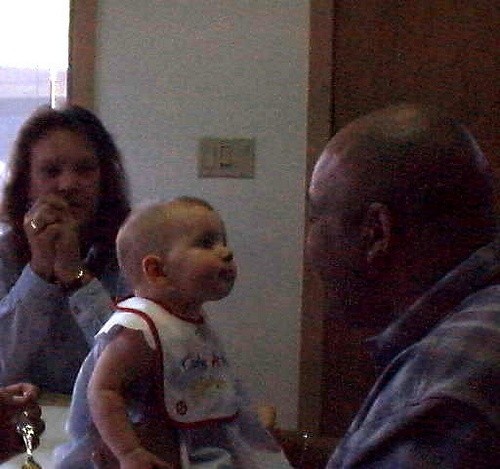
29;219;41;233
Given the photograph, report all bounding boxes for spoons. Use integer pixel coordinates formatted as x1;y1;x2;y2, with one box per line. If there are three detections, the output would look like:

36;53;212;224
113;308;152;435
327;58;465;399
18;399;43;469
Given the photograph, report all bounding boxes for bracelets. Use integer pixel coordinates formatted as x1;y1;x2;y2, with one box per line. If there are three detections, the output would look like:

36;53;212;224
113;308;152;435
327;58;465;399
64;268;84;285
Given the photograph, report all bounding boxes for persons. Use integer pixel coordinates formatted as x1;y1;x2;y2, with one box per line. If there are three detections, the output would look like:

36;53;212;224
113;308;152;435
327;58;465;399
74;197;277;469
0;103;145;399
0;383;45;465
260;101;499;469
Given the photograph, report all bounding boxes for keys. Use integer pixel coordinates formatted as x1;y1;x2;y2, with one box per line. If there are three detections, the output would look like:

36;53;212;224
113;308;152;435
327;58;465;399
15;404;36;462
